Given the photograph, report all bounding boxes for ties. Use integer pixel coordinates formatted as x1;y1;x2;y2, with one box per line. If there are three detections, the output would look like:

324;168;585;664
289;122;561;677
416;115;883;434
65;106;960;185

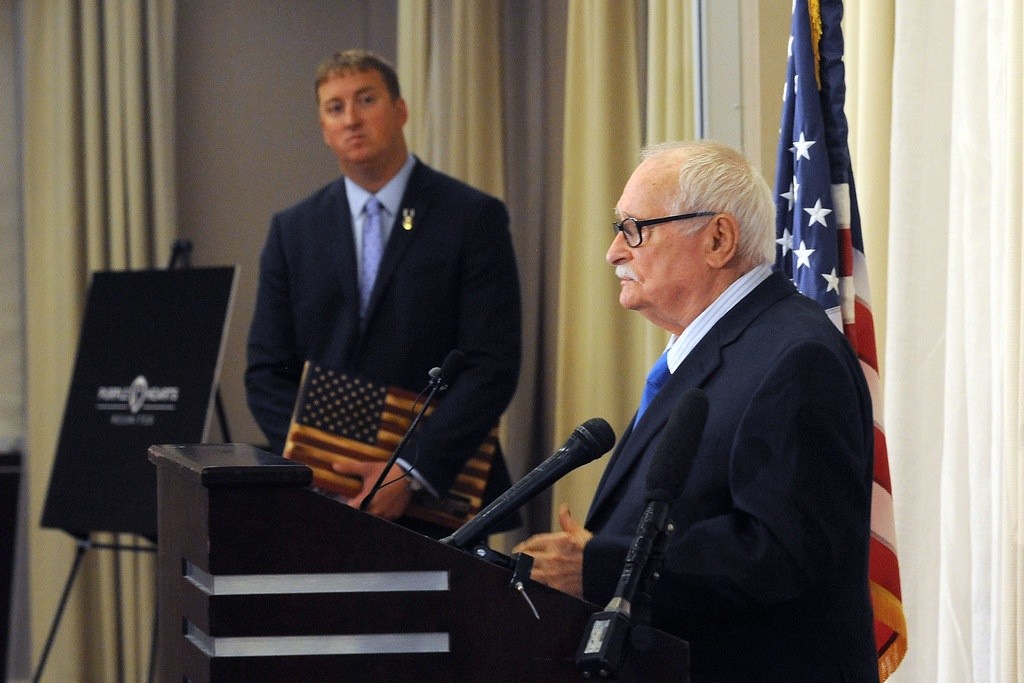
631;348;672;434
357;197;385;316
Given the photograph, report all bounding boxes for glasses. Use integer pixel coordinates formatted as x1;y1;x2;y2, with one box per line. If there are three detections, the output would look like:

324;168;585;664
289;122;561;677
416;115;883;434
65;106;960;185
612;208;720;248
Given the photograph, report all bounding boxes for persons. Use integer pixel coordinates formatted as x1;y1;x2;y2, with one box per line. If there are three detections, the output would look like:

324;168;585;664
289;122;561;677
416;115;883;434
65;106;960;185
510;139;877;683
244;48;525;551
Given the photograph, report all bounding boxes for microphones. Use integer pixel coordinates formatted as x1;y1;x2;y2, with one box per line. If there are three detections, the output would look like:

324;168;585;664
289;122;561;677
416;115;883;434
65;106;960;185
430;349;469;386
574;389;711;683
440;418;616;548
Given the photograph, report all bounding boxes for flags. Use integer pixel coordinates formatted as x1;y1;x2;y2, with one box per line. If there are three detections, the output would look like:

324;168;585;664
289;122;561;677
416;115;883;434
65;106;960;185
771;0;910;683
